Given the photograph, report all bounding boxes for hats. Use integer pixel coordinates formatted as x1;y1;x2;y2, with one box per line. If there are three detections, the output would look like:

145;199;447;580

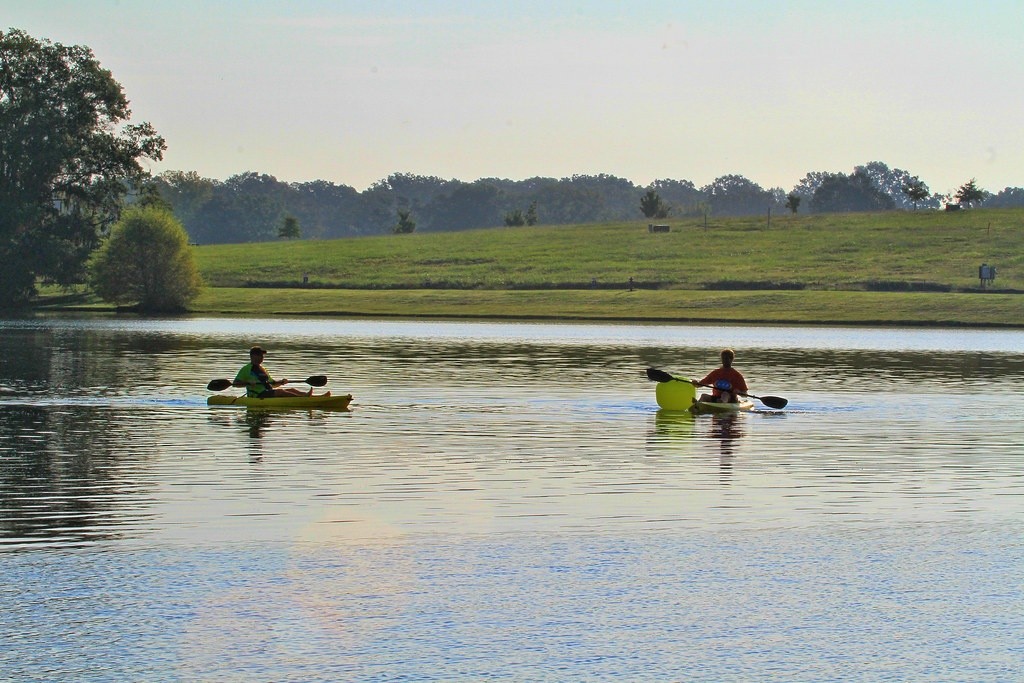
249;346;268;355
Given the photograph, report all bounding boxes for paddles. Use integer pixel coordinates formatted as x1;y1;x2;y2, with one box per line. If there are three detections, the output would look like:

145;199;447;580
647;367;788;409
207;376;328;391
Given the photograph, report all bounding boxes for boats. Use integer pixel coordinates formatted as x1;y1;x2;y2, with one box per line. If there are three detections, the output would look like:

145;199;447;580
207;394;354;412
688;397;756;417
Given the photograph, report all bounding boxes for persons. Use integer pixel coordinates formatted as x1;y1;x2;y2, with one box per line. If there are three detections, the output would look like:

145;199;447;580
231;345;332;400
689;347;748;408
